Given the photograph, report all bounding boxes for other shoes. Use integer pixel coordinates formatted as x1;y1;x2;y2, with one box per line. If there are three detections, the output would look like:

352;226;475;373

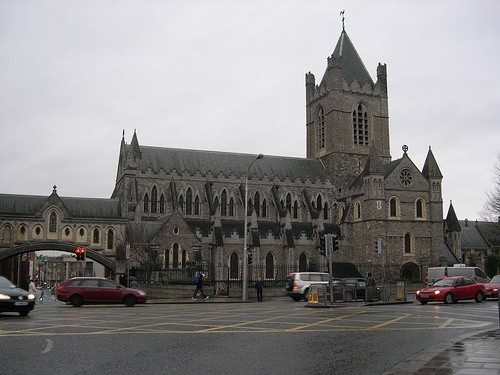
205;296;209;301
192;297;198;301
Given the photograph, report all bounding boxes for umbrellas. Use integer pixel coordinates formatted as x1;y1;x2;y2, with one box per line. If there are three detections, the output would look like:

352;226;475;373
125;276;138;280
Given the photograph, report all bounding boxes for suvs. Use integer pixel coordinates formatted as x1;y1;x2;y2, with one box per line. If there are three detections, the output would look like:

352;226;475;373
284;272;334;301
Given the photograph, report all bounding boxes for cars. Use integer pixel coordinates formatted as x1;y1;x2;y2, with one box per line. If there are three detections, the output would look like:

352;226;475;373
416;277;485;305
326;277;390;302
485;275;500;301
0;275;36;316
56;277;147;308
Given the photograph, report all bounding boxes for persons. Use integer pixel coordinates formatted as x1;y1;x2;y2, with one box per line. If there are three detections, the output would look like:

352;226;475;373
24;275;37;305
254;278;265;303
192;273;209;301
367;273;375;300
130;280;139;290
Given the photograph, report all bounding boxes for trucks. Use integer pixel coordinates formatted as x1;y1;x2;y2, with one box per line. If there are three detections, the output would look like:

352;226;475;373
426;267;491;287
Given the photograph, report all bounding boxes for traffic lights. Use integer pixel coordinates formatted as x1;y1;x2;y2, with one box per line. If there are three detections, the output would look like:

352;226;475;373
331;235;339;252
320;235;328;257
76;248;81;260
375;240;383;255
81;249;86;260
247;253;253;266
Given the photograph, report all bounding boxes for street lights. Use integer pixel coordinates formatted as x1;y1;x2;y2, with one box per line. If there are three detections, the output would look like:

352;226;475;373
243;153;263;301
